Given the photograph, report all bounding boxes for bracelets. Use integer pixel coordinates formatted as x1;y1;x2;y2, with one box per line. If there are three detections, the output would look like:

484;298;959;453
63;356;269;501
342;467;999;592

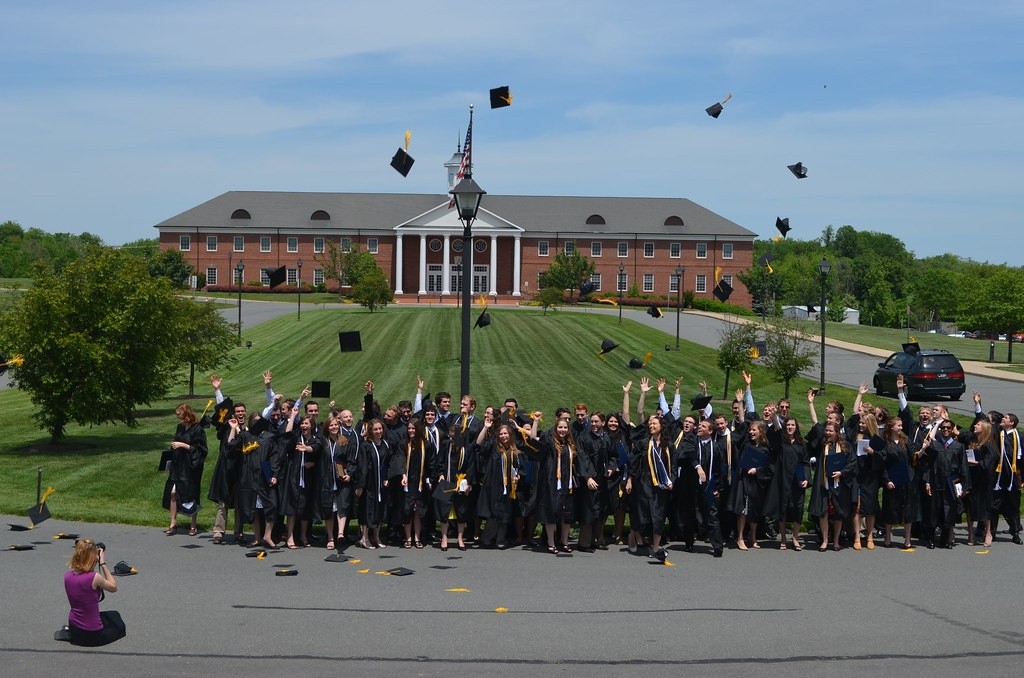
100;562;106;566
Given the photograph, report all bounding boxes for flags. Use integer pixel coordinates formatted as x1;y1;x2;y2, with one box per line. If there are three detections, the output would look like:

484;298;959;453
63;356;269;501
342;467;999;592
449;118;472;208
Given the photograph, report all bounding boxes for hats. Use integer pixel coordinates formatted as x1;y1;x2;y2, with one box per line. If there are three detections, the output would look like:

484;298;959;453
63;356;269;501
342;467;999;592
597;339;620;361
579;279;618;306
758;251;776;274
489;86;512;110
648;548;673;566
647;304;663;318
712;266;734;304
690;393;714;411
473;295;490;330
266;265;286;289
704;93;733;119
749;341;767;359
389;130;415;177
339;330;362;352
774;216;792;245
311;380;331;399
245;549;416;577
787;161;809;179
7;486;137;577
626;352;651;369
901;336;921;353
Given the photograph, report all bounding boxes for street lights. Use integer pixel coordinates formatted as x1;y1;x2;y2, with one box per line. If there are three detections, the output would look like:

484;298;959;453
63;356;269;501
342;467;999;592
817;257;833;395
296;257;303;319
674;263;685;351
456;258;462;308
618;262;625;324
449;170;487;396
237;259;245;348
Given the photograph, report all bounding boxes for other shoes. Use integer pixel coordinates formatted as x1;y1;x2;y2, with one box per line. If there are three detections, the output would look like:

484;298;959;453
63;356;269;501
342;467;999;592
54;629;71;640
166;523;1023;558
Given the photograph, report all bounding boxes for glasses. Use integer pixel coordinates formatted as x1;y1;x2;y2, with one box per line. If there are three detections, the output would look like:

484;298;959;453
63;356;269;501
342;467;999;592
942;426;951;429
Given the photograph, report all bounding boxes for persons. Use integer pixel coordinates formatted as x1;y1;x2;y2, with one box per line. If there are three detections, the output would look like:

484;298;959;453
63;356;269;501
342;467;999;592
211;369;1024;554
54;539;126;647
163;404;209;535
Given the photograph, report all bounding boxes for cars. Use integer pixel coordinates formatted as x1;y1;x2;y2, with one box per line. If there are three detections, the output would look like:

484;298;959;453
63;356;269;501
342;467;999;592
946;331;972;338
967;330;992;339
872;348;966;400
928;329;947;334
999;332;1024;343
